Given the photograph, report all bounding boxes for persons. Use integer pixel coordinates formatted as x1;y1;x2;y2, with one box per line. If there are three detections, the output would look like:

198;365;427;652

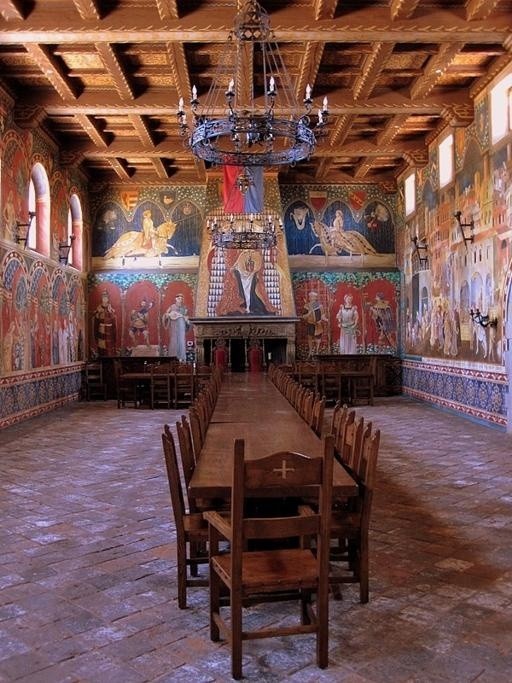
128;297;155;347
370;291;397;346
162;293;191;362
330;208;343;235
2;310;84;371
139;208;159;246
405;293;504;363
92;288;117;357
336;292;360;355
301;288;330;360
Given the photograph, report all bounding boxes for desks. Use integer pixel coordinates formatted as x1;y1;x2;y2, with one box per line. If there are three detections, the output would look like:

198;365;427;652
188;424;359;595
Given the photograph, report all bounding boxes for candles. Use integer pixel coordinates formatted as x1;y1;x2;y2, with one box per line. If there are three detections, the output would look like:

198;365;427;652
207;212;285;234
177;77;330;124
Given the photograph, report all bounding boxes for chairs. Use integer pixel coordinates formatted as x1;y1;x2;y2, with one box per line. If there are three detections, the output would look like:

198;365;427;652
160;423;228;610
286;356;377;409
308;421;380;603
85;361;109;401
202;435;335;680
339;411;365;473
113;359;227;410
188;365;356;466
175;415;231;557
